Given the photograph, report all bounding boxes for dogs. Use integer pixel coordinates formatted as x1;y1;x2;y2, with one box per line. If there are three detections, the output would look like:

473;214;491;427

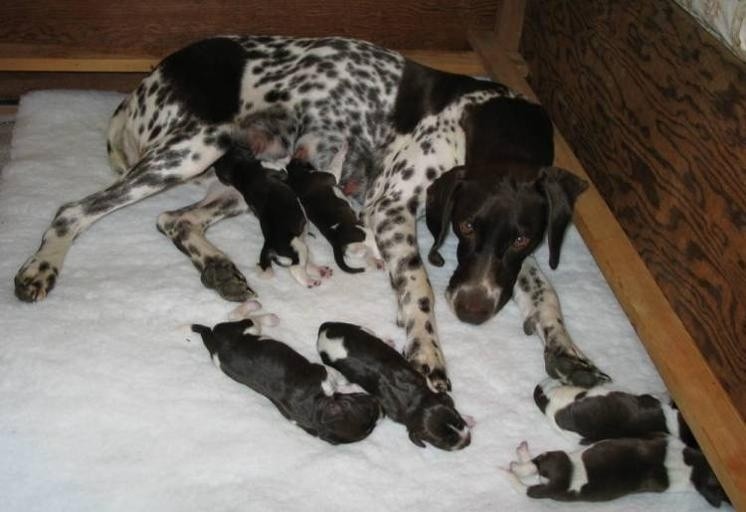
191;300;385;445
13;34;613;394
534;377;700;449
286;158;385;274
214;150;333;289
509;431;731;508
317;321;476;452
